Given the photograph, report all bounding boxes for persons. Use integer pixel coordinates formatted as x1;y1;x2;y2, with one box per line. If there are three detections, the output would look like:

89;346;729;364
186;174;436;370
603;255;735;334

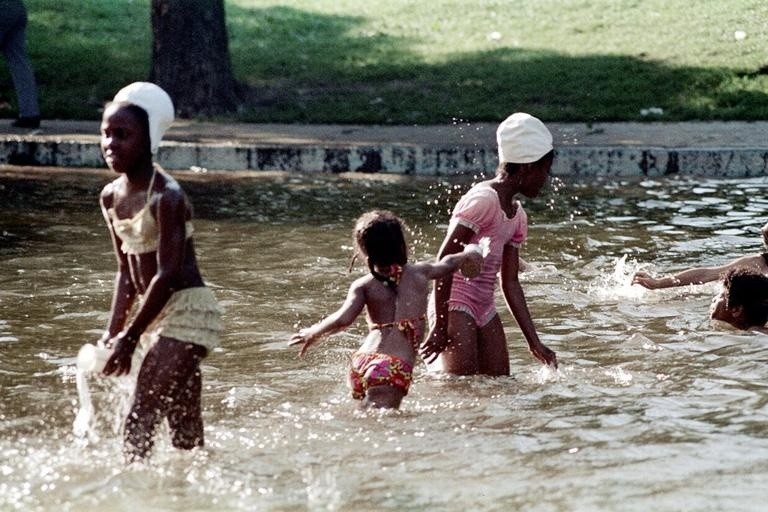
96;80;224;464
286;208;483;414
1;0;43;132
418;110;559;383
709;265;768;330
630;221;768;292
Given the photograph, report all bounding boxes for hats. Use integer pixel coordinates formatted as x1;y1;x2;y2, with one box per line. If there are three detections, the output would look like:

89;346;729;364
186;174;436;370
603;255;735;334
114;80;176;155
496;112;553;164
